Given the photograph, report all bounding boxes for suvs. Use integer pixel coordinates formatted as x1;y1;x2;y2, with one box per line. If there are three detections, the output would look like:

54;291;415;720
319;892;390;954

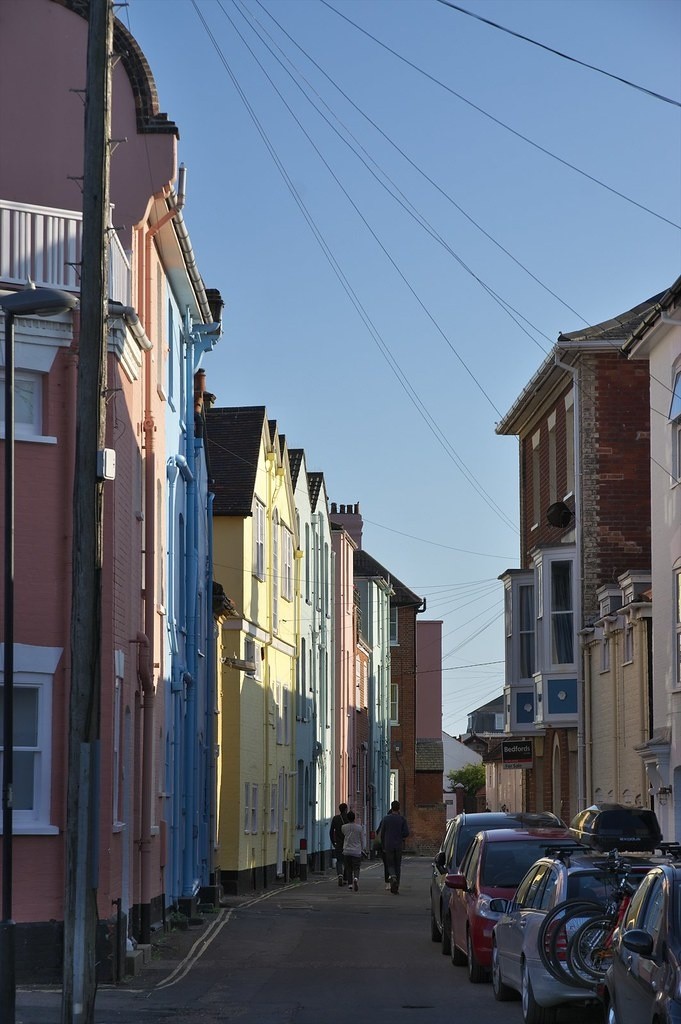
489;803;681;1024
429;811;569;956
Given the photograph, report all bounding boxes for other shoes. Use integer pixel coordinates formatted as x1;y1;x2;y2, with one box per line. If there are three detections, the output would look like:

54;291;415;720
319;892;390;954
353;877;359;893
343;880;348;884
339;877;343;887
391;878;398;893
348;885;353;890
386;886;390;890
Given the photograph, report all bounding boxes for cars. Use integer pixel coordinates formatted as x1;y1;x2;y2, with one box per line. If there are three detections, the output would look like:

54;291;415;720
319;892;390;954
602;862;681;1024
445;828;596;983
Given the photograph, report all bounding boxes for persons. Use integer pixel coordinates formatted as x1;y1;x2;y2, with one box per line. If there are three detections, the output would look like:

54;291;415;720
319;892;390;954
330;804;348;886
341;811;364;892
377;801;410;894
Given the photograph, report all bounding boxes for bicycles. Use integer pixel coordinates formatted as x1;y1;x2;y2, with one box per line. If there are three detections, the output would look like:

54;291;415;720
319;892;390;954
537;846;650;992
